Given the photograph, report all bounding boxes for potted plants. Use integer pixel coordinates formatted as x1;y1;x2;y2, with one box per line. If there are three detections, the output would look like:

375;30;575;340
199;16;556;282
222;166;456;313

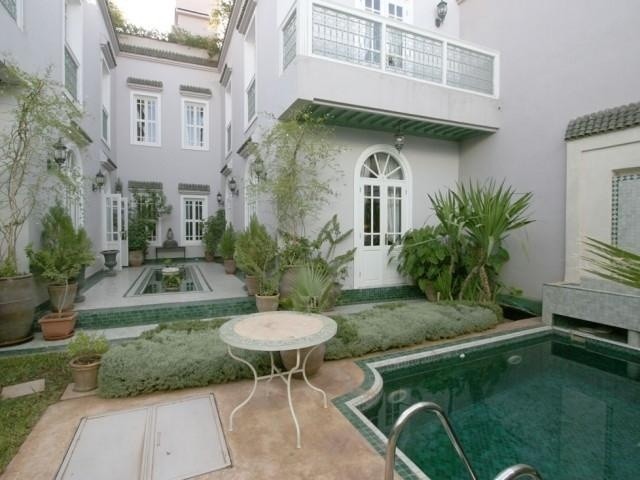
0;101;110;392
245;106;358;312
233;212;278;295
216;221;238;274
279;261;335;378
202;210;227;262
113;182;173;267
387;177;537;304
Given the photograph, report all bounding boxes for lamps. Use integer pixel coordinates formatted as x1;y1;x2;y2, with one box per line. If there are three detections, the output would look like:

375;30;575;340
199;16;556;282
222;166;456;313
393;120;405;155
435;0;448;27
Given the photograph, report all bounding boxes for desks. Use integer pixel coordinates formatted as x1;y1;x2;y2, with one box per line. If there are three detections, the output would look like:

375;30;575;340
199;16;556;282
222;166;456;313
218;310;338;449
